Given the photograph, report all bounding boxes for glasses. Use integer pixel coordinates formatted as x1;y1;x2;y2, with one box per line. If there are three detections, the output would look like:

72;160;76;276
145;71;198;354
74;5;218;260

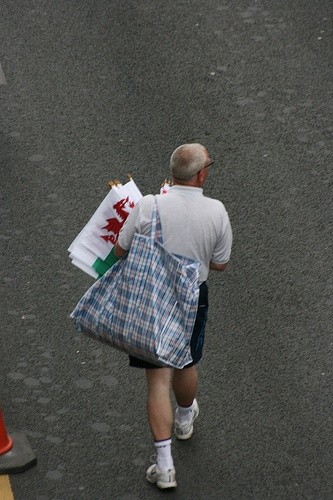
197;160;215;175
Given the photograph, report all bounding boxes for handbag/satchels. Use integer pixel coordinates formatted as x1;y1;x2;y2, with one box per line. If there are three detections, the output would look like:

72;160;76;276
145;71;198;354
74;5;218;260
69;199;200;370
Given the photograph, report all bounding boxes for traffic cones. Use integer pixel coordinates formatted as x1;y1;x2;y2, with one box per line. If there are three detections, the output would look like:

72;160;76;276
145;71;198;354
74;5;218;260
0;401;38;475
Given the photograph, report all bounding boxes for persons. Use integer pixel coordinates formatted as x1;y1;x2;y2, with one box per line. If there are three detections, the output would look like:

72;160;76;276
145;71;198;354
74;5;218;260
115;142;232;488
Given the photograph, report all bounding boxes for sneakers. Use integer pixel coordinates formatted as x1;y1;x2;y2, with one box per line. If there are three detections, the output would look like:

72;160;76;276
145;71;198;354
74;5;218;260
147;464;177;488
173;398;199;439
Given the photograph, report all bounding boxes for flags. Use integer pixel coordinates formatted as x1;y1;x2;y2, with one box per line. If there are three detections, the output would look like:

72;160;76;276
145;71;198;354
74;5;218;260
67;180;170;281
